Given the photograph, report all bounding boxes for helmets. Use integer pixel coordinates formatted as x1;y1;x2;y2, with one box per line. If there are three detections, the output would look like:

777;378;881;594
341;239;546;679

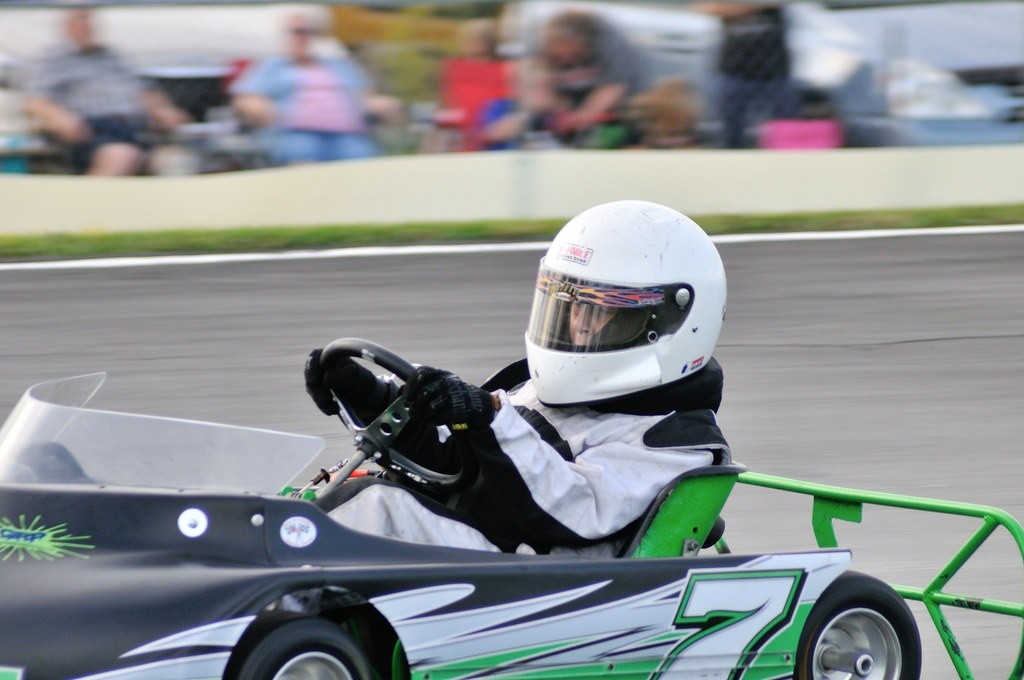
525;200;728;408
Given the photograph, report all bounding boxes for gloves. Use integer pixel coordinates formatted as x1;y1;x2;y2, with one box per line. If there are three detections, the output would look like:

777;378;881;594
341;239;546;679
304;348;387;426
398;366;501;434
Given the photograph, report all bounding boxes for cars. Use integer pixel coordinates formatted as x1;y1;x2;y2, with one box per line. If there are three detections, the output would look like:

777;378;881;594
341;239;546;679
493;0;1023;146
0;0;337;174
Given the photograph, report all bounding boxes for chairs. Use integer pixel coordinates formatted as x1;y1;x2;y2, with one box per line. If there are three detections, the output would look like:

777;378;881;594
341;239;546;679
622;460;747;557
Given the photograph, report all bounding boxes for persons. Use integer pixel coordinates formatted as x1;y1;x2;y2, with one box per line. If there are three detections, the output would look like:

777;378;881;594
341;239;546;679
686;0;794;147
436;17;532;151
522;11;647;148
307;201;729;556
11;4;200;177
228;14;405;164
